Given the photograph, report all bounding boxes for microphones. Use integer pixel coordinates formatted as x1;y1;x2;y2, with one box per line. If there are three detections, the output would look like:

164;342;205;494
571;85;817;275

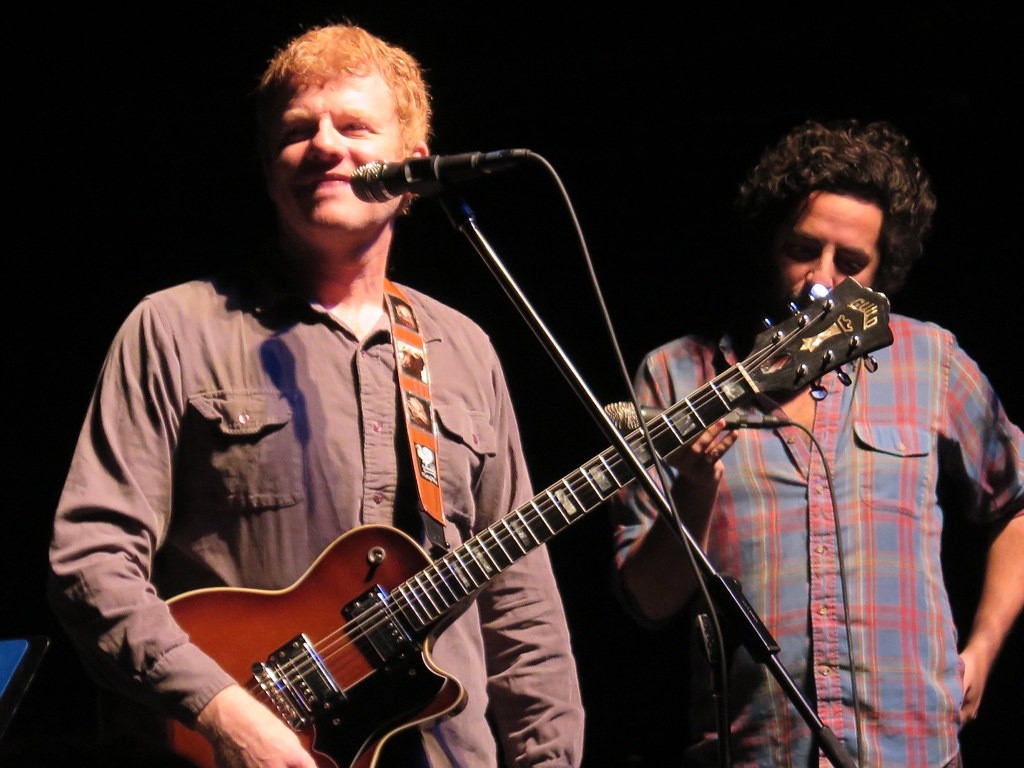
602;404;794;438
348;149;532;204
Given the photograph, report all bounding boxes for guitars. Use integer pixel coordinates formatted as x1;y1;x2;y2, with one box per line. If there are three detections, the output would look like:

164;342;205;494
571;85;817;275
93;272;895;768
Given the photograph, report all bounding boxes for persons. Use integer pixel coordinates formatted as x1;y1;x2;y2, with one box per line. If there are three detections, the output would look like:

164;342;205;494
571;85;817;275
46;25;587;768
612;118;1024;768
